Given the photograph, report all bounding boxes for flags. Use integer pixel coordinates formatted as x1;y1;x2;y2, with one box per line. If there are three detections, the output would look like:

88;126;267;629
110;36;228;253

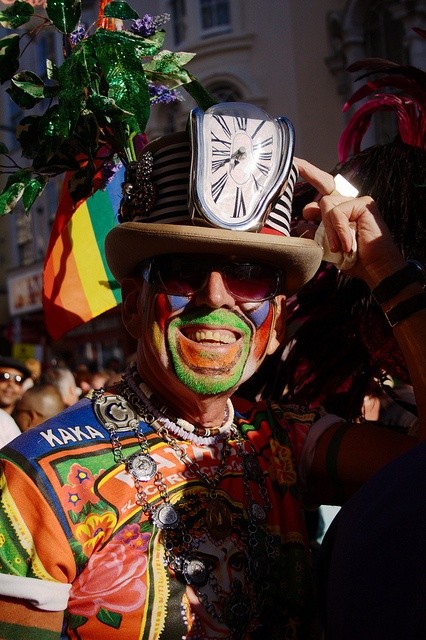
39;130;124;340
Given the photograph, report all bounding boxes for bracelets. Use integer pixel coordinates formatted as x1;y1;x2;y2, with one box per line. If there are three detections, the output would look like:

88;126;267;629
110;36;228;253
385;284;426;327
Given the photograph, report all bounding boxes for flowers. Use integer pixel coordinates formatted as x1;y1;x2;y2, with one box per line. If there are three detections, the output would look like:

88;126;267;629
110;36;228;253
0;0;187;219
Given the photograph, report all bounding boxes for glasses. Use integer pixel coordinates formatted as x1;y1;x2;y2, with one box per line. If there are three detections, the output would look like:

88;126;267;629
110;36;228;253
0;372;24;383
142;257;285;302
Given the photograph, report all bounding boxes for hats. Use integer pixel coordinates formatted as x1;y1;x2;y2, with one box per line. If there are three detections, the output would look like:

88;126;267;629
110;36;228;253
105;102;323;293
0;359;28;378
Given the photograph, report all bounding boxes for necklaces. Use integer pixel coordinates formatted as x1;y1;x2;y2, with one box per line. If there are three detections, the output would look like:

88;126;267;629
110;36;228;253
123;364;233;446
88;374;278;640
130;359;235;437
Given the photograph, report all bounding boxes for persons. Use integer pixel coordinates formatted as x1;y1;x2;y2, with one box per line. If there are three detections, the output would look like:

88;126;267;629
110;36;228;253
0;360;32;406
0;255;426;640
17;383;64;434
259;139;425;436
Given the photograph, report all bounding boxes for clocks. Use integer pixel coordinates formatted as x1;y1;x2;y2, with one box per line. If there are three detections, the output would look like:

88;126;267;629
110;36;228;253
188;101;295;231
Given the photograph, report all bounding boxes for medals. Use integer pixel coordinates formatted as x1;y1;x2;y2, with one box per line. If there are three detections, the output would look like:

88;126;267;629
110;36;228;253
119;379;234;540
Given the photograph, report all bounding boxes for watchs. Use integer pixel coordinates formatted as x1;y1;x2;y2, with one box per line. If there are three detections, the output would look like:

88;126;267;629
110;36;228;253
369;258;426;307
